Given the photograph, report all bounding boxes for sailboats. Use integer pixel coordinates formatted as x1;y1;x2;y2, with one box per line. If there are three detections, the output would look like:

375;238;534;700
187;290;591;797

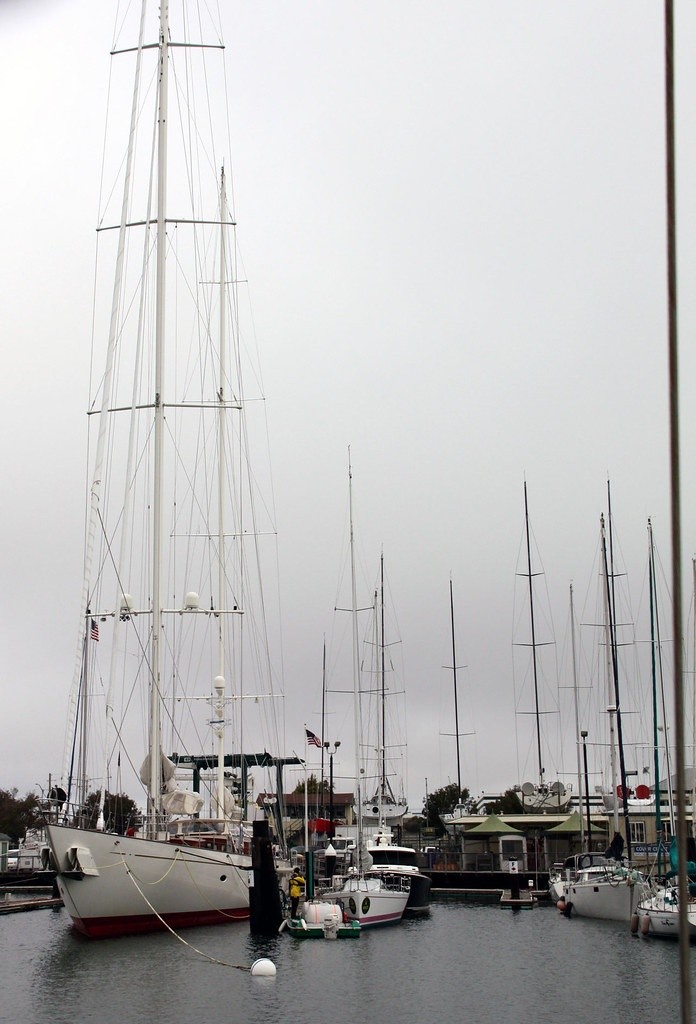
0;0;696;951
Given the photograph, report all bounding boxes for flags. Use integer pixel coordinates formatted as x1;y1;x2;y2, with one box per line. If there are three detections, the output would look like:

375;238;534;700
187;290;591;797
306;729;321;748
91;618;98;642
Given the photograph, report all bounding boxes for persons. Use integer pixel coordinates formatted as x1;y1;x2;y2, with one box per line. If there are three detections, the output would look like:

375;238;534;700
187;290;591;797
616;856;629;869
672;888;679;905
272;842;280;857
656;888;663;902
126;825;136;837
289;867;305;920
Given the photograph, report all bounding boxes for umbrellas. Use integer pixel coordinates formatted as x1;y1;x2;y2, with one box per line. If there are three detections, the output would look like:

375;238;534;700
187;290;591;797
546;811;608;855
465;814;524;872
